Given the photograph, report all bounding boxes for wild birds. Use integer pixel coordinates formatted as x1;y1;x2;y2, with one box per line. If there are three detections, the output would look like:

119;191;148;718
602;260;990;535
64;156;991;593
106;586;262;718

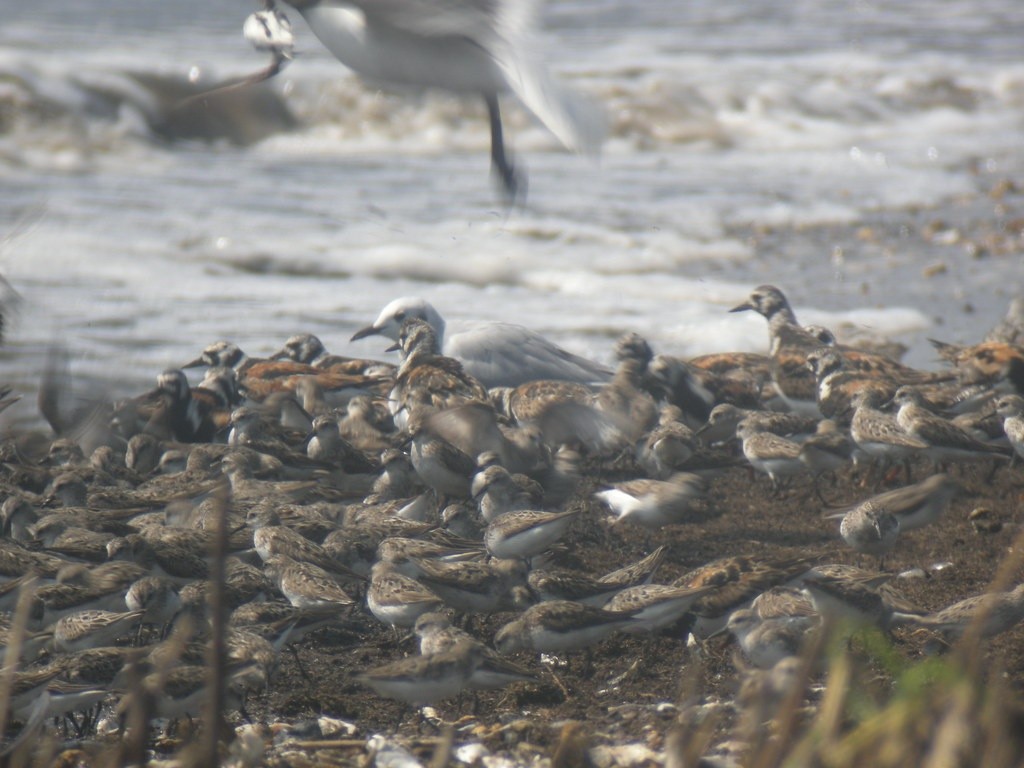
0;283;1023;734
178;1;608;216
